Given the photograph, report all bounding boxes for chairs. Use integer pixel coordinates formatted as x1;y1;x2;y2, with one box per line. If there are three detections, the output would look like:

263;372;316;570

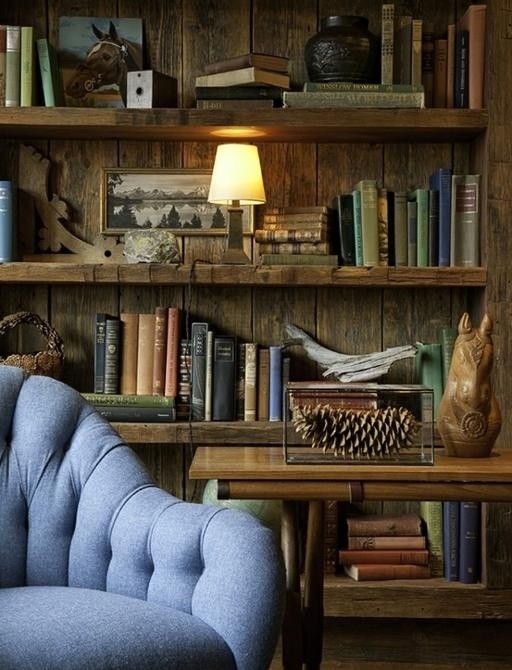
0;366;284;670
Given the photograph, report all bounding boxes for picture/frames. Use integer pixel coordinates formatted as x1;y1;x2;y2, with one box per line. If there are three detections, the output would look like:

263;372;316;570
100;166;254;234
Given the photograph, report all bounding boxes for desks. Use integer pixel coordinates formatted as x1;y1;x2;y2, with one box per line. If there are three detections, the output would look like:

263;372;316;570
188;447;511;670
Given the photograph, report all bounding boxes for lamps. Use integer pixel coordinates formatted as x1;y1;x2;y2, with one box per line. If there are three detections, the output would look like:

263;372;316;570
208;143;266;264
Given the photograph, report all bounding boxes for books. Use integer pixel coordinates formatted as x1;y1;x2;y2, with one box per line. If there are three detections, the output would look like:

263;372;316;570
254;166;482;268
94;305;290;425
439;327;458;394
7;26;21;107
193;0;484;109
338;498;481;583
1;24;6;106
19;26;34;107
0;181;20;263
36;38;65;107
414;344;443;424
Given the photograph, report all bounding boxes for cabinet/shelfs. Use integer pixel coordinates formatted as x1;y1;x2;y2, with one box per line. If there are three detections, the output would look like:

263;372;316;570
0;103;485;444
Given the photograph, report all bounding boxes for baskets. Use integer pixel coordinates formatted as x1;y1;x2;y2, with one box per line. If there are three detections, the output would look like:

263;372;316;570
0;312;66;381
